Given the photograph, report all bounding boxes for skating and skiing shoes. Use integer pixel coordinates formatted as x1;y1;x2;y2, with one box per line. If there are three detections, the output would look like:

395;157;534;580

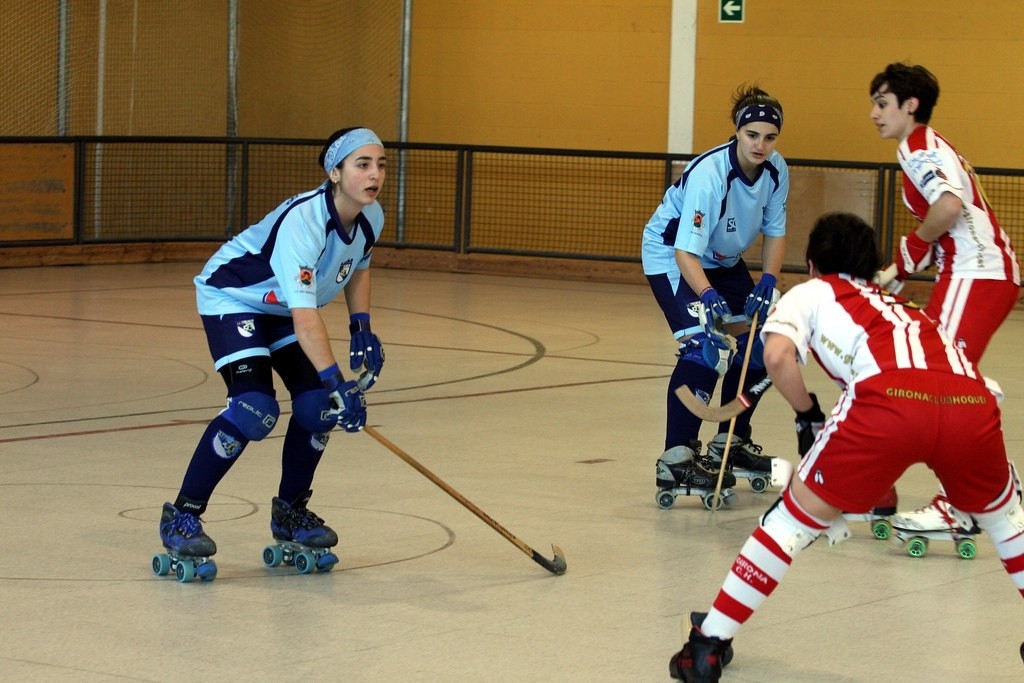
706;433;778;493
262;489;339;574
655;441;737;511
840;484;982;559
151;502;217;584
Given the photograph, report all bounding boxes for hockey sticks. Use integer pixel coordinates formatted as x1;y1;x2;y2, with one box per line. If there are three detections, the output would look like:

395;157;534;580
705;311;762;534
675;264;903;426
364;423;569;576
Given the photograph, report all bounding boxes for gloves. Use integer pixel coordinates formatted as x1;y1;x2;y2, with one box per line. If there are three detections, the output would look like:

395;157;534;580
349;312;386;392
699;288;732;350
795;393;825;458
744;273;777;330
318;362;368;433
877;227;933;296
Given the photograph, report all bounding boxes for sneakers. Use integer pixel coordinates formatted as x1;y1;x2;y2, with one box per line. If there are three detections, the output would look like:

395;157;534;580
668;612;733;683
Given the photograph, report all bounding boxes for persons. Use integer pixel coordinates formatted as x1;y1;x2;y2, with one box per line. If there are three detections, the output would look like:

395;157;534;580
672;211;1024;683
870;61;1021;558
641;86;790;509
151;126;386;582
